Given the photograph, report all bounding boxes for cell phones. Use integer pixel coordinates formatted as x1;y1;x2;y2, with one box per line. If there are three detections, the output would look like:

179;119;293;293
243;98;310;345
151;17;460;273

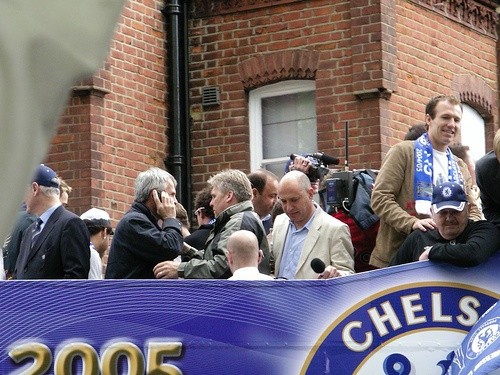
157;191;162;202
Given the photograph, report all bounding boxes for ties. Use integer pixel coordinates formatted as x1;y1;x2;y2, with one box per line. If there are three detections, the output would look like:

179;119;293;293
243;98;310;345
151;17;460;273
31;218;44;249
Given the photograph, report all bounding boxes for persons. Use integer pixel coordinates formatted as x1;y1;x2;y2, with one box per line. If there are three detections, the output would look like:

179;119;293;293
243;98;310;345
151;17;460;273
0;94;499;280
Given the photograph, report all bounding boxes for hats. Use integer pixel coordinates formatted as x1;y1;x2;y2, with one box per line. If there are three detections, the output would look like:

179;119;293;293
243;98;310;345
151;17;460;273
30;164;61;188
80;208;114;235
431;182;467;214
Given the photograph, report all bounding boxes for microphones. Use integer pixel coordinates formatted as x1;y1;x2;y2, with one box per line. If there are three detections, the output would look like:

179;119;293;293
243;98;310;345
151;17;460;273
312;153;339;165
310;258;328;276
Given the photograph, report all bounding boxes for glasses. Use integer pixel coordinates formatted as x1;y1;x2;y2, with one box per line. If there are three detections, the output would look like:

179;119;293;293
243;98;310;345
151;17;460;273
195;207;205;215
